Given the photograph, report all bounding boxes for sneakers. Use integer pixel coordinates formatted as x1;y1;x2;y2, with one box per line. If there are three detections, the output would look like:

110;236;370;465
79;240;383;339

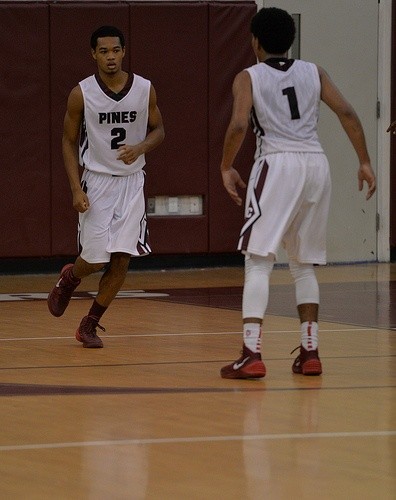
48;264;81;317
290;344;322;375
221;350;266;378
76;315;106;348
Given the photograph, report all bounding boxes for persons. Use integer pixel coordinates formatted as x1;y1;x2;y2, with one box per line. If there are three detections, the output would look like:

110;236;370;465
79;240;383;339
220;7;376;379
47;25;166;350
